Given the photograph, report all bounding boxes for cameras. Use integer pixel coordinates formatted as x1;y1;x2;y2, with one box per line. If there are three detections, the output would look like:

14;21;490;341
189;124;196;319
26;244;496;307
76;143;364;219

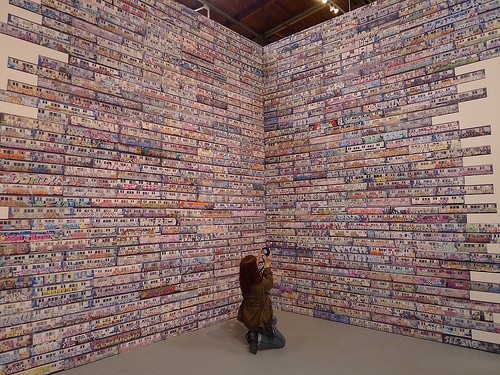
261;247;271;257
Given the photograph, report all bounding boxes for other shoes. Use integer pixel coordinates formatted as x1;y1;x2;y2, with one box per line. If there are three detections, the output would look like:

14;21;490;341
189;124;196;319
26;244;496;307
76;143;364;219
246;330;257;354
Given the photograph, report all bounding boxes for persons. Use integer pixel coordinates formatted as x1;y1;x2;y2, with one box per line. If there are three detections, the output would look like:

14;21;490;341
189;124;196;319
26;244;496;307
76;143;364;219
237;247;286;355
